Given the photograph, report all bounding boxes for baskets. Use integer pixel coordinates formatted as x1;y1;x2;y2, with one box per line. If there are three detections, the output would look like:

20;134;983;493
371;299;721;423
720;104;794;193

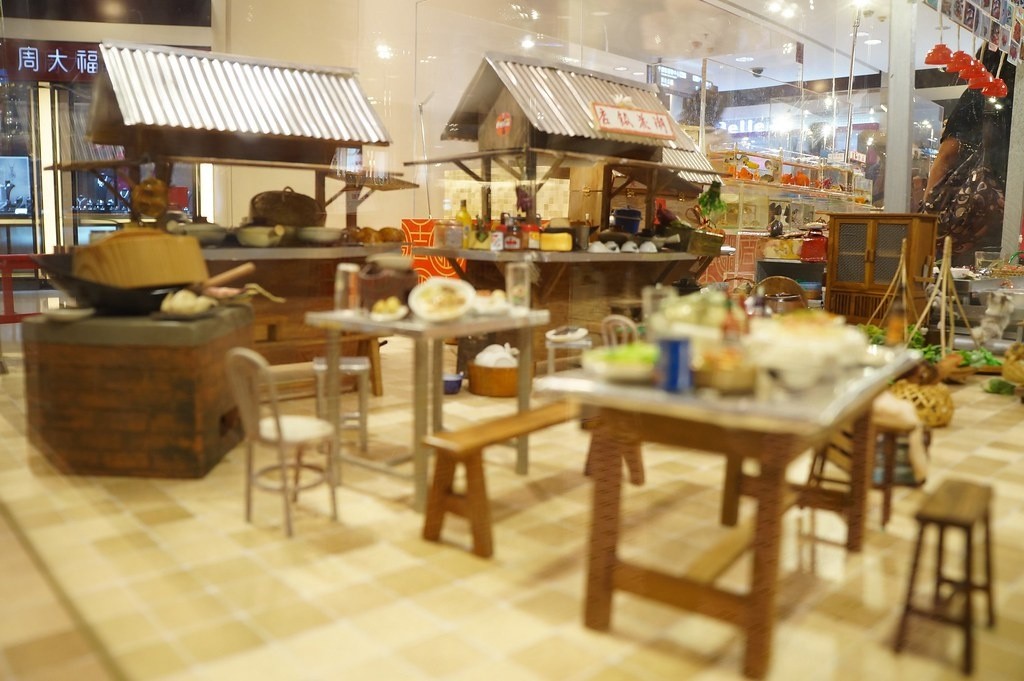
249;186;324;230
1002;344;1024;386
992;259;1023;279
934;358;976;385
724;277;757;299
465;359;533;397
893;379;954;427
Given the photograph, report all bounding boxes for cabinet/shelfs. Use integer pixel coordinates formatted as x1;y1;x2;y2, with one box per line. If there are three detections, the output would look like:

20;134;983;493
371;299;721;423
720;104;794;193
816;211;941;348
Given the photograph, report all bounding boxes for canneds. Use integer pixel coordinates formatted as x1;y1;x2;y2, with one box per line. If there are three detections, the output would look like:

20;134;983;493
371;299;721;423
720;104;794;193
656;336;690;394
491;224;540;251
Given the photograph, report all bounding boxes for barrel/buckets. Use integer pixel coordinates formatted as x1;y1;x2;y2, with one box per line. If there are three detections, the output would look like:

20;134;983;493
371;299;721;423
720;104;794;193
921;324;950;347
610;204;643;235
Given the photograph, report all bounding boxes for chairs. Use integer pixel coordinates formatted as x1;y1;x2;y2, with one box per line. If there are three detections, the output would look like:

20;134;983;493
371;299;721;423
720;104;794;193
222;345;339;538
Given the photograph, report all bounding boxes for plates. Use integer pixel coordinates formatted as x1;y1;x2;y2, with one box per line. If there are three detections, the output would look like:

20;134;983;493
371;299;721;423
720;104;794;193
39;307;95;323
473;343;517;369
544;325;588;344
578;343;662;384
368;304;409;323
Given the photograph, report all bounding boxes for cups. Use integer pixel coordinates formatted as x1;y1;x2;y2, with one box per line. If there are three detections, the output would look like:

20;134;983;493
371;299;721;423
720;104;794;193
504;262;531;319
656;336;692;395
761;291;805;321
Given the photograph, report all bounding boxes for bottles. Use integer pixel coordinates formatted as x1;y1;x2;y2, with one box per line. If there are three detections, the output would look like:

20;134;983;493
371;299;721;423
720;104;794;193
454;199;473;250
584;212;591;227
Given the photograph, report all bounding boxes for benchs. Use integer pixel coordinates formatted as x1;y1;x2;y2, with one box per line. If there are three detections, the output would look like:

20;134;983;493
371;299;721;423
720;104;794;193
256;331;395;397
421;397;645;559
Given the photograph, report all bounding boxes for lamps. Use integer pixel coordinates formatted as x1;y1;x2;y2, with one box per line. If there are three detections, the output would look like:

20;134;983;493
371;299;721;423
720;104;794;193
923;0;1009;97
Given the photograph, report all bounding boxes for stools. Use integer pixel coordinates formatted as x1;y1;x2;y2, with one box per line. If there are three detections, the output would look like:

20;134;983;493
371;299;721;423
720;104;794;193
801;389;918;527
891;476;996;676
309;355;373;461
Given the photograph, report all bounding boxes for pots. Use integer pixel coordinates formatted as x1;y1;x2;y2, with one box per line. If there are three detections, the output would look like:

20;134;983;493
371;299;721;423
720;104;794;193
25;249;256;320
640;233;681;249
249;186;328;243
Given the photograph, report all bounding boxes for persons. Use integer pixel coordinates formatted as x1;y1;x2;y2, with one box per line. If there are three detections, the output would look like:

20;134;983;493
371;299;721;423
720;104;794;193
919;38;1016;210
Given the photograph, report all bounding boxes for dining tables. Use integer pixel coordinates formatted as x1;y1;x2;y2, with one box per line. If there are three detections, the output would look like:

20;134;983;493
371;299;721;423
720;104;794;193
537;340;928;680
304;301;551;512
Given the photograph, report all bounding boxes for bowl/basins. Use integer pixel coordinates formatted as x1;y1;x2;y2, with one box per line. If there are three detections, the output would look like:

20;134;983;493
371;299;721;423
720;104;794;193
442;373;463;395
297;225;343;246
465;357;534;398
949;268;970;279
234;224;286;248
165;222;228;246
585;240;658;254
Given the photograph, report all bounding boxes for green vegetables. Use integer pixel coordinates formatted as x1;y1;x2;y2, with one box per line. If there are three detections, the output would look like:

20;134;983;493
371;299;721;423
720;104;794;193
953;347;1003;367
857;322;888;345
906;323;952;363
